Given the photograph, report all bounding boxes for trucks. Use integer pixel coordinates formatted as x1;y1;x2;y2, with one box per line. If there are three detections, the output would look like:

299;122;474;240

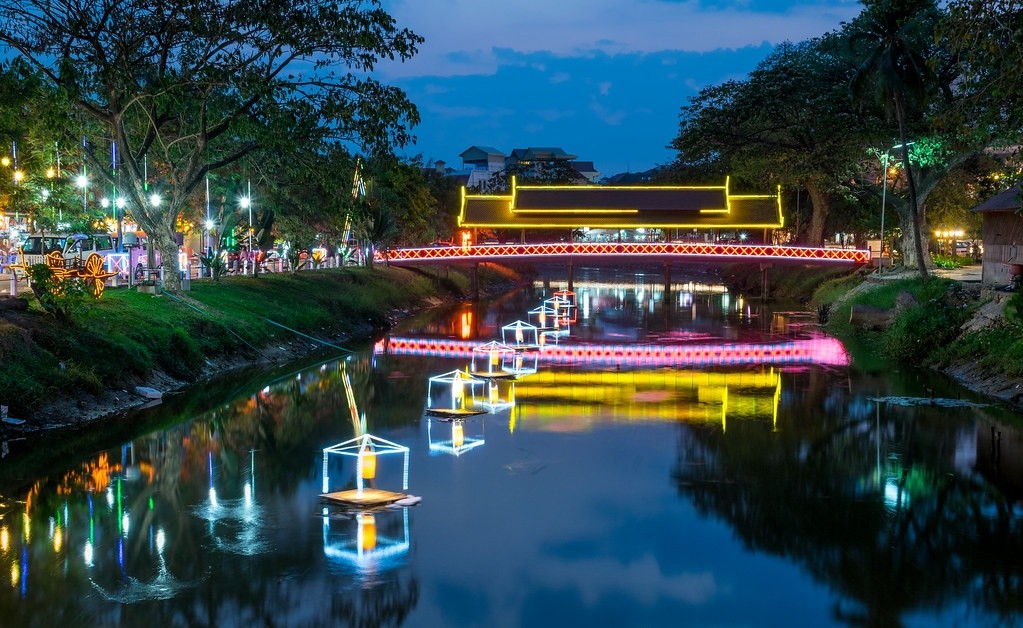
15;228;189;281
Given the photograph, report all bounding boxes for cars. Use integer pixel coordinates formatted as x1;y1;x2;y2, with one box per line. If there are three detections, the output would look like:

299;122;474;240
937;239;984;257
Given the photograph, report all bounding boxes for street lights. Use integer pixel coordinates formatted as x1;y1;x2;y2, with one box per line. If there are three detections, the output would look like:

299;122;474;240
47;169;54;220
76;174;86;214
240;196;252;253
15;170;23;221
878;141;915;274
102;197;125;222
204;220;213;258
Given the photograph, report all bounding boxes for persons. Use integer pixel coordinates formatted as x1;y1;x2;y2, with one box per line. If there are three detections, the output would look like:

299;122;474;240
240;248;248;272
221;249;229;269
966;242;978;258
0;244;18;264
